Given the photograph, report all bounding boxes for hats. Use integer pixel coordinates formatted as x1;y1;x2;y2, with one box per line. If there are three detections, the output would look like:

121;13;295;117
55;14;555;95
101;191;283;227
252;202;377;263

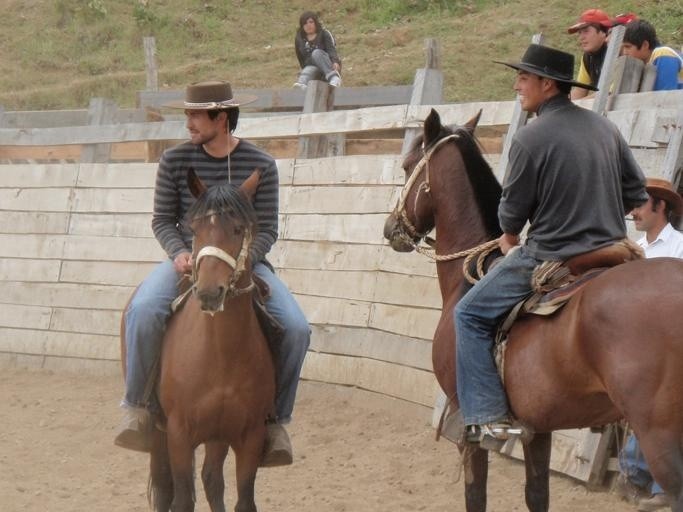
644;177;683;216
600;13;639;27
567;8;613;35
491;44;600;91
161;79;260;111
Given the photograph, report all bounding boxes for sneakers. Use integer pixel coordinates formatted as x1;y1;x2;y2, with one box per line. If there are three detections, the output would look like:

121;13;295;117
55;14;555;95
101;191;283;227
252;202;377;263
290;83;308;89
326;74;343;88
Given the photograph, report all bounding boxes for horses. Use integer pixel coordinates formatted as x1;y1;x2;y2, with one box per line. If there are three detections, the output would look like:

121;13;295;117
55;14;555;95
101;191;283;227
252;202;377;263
382;107;683;511
119;165;275;511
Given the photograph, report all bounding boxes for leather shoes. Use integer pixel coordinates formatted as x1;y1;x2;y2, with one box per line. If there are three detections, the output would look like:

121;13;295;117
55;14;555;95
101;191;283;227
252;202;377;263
616;471;649;507
465;424;507;451
637;491;671;511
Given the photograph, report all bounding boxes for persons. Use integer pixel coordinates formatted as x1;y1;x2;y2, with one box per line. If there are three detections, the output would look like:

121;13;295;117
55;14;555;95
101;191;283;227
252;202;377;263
567;9;618;98
105;80;312;468
455;44;647;443
293;11;342;94
619;17;682;93
618;177;682;512
